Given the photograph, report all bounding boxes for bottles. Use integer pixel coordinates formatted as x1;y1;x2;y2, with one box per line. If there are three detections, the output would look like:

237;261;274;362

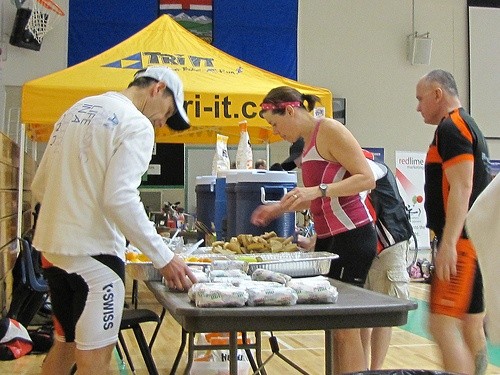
176;209;186;229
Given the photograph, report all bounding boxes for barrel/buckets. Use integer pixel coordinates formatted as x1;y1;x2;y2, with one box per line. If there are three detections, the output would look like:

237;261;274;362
196;168;298;246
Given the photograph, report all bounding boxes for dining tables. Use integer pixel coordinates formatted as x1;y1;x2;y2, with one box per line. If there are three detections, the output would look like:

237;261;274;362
131;263;418;375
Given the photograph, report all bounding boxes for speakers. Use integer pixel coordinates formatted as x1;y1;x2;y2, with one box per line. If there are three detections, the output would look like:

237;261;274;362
9;8;48;51
410;37;433;65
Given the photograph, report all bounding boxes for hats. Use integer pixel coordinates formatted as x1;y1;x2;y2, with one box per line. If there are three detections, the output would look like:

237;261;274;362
132;64;191;131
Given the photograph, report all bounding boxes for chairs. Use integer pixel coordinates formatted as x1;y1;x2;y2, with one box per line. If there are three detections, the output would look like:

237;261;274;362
71;309;160;374
18;240;51;330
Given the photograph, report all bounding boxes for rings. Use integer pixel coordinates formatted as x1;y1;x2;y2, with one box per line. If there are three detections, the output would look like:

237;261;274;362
292;194;298;199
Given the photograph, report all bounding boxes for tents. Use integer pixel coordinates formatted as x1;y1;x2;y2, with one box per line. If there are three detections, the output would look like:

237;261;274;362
15;13;333;257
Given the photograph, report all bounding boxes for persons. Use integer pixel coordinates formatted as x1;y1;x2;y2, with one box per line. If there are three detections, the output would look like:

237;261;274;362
282;136;413;371
250;85;376;375
254;159;267;170
31;64;196;375
416;70;493;374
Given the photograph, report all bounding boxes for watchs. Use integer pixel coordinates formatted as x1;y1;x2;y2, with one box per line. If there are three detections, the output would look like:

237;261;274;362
318;182;329;198
